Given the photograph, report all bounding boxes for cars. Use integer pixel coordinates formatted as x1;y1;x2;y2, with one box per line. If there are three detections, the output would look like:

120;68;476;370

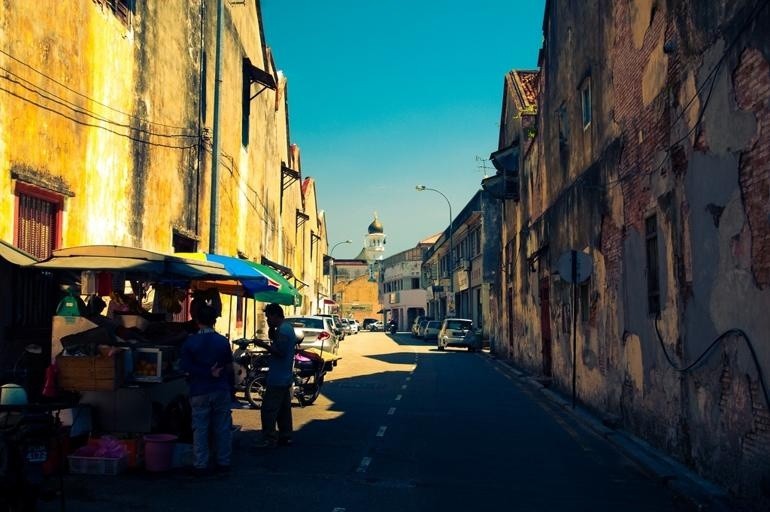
285;316;342;371
438;318;479;353
363;318;385;331
418;320;428;337
425;321;445;340
318;314;361;341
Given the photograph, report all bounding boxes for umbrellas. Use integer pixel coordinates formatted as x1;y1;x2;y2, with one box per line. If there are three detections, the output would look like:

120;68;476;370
172;252;267;296
196;255;303;307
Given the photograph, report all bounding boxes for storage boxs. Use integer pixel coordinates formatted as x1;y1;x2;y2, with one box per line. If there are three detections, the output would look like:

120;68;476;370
55;348;121;393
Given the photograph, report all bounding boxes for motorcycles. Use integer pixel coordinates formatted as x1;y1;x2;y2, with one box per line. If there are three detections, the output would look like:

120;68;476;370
230;329;329;410
390;324;396;335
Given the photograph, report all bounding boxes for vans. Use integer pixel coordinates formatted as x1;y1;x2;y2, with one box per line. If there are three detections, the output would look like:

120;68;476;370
412;316;432;336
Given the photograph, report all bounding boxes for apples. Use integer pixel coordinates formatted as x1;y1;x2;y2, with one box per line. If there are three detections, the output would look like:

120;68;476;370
135;361;156;376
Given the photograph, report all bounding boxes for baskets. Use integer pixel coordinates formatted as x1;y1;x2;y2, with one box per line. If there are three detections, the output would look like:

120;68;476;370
67;455;125;478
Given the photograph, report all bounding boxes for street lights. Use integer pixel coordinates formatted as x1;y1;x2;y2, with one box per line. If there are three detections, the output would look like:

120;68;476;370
331;241;352;256
417;185;454;292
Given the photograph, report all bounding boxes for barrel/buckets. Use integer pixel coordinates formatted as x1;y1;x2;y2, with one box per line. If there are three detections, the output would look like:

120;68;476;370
142;432;179;471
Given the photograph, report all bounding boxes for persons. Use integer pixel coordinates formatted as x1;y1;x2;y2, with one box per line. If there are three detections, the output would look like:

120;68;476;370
179;304;235;473
251;302;296;446
